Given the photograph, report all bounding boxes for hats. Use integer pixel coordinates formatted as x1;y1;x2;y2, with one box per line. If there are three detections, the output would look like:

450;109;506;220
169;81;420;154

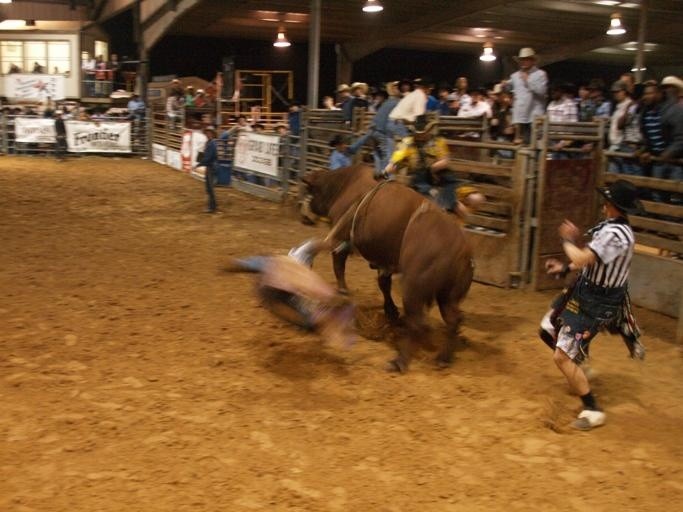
584;77;606;90
510;47;542;66
609;80;627;93
593;178;649;218
334;76;516;103
169;78;208;98
327;132;350;147
657;74;683;96
201;112;292;138
404;113;442;136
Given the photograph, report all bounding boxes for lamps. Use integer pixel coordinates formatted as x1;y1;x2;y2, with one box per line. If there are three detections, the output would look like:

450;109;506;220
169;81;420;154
478;41;496;62
360;0;383;14
629;48;647;72
271;13;292;48
605;5;627;36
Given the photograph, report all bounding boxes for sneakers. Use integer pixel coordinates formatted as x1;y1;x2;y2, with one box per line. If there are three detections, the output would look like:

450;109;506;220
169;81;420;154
571;408;607;431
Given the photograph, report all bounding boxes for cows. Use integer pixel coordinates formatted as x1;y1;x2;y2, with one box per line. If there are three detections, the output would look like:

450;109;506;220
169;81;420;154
296;164;473;375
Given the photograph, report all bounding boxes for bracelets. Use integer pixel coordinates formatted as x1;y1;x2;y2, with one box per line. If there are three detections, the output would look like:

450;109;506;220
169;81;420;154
561;261;571;275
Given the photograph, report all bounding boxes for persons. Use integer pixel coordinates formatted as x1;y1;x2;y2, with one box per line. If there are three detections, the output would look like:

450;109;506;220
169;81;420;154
538;178;648;431
195;126;218;213
507;48;547;147
547;73;682;241
1;54;145;156
167;80;299;187
323;77;515;235
222;240;358;352
